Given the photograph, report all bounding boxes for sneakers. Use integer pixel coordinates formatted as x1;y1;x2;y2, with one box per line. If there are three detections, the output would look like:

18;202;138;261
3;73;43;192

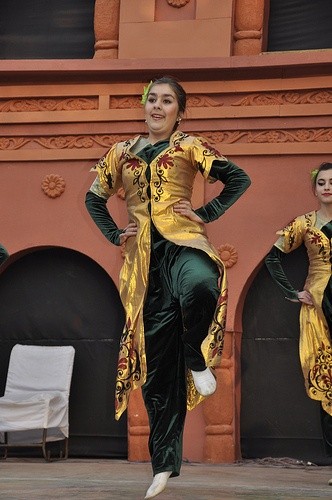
190;367;217;398
142;472;173;500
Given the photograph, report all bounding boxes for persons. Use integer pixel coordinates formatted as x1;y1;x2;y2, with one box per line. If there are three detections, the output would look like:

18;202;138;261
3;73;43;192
262;161;332;486
83;76;248;499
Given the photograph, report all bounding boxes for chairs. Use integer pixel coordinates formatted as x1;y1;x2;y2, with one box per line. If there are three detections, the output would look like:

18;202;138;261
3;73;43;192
0;343;76;462
